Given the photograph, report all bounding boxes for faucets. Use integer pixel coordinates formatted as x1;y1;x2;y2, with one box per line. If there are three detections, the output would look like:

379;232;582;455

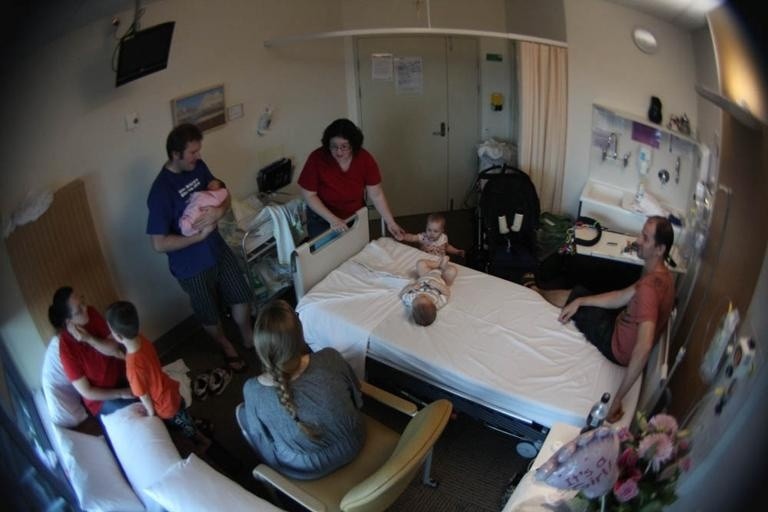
607;133;618;155
633;184;647;200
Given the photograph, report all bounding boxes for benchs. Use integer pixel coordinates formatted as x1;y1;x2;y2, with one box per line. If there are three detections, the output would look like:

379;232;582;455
36;387;209;512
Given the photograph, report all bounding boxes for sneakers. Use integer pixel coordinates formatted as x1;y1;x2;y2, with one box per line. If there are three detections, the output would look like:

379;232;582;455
191;367;233;401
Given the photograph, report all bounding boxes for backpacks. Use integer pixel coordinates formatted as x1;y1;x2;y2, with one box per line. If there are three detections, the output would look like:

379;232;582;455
466;172;540;280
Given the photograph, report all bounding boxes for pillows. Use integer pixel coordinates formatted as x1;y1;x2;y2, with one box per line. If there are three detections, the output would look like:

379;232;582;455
100;401;183;512
52;422;143;511
142;452;286;512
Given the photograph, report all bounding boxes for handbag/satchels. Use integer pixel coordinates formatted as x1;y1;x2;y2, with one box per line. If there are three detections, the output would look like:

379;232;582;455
254;157;292;195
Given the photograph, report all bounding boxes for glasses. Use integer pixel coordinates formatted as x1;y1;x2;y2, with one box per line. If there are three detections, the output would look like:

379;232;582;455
65;294;86;318
329;144;352;151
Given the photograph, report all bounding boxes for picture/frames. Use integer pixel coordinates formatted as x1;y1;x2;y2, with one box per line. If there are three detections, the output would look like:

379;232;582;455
170;82;226;135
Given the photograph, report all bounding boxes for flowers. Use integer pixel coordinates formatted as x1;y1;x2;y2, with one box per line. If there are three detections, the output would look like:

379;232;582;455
570;408;691;512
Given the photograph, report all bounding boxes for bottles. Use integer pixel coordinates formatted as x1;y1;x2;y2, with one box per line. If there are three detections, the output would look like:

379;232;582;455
579;392;611;435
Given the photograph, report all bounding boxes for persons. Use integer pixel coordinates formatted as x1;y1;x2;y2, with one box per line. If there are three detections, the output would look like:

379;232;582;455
105;300;218;460
241;297;366;482
144;123;256;376
177;177;229;236
40;331;102;436
399;255;458;327
395;212;464;257
47;284;217;436
296;117;404;253
521;214;677;425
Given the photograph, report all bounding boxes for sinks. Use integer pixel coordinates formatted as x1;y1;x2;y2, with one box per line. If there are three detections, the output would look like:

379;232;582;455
579;182;689;247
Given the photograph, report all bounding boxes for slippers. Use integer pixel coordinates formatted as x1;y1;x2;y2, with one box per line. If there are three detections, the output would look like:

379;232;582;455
520;271;538;288
225;353;244;373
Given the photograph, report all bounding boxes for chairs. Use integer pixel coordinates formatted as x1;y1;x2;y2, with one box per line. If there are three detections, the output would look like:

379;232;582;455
252;379;453;512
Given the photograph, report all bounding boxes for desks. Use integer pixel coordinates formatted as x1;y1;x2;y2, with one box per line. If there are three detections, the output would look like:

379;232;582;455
576;226;689;304
502;422;633;512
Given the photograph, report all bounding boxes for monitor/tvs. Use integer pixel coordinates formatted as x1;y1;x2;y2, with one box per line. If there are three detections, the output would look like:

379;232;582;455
116;21;176;88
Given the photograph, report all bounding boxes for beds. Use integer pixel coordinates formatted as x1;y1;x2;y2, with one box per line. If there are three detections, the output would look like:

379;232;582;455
290;208;673;461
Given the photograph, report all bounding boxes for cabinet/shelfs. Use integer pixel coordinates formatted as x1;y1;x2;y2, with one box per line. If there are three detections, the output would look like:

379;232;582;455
215;191;306;312
